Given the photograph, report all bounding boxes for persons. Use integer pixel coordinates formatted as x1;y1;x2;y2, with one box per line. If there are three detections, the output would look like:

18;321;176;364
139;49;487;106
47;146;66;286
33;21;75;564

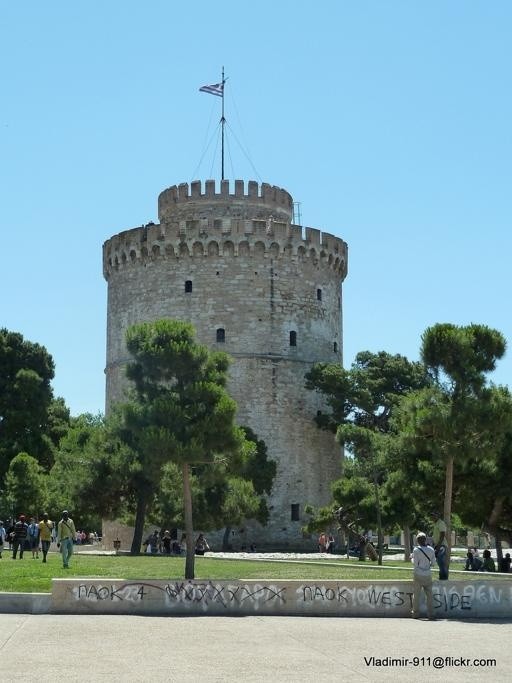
319;511;512;621
143;530;210;555
0;509;98;569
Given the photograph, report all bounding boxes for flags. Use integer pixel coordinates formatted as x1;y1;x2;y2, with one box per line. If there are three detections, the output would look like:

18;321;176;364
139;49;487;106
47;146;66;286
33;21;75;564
199;82;223;97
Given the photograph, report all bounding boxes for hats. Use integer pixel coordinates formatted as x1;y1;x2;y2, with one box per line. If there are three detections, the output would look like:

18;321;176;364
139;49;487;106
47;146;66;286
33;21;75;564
416;532;425;538
